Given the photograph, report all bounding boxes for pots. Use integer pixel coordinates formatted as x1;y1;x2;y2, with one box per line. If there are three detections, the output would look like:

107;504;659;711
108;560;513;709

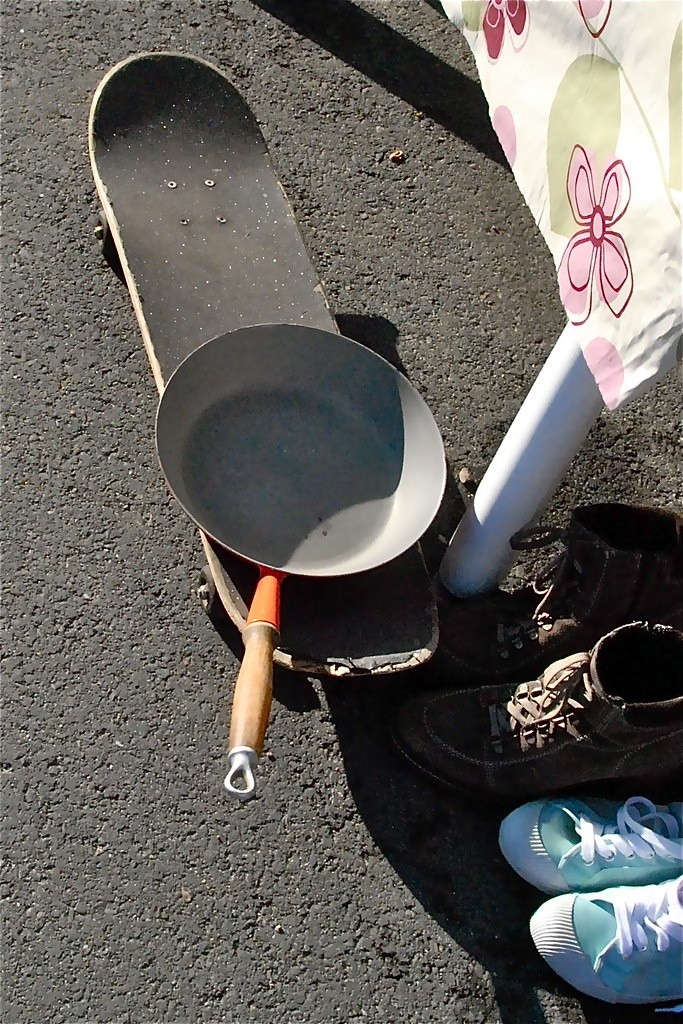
153;323;450;795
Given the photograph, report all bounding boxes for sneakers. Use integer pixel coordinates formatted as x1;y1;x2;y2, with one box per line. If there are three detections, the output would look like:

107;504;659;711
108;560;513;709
498;793;683;1016
383;504;682;807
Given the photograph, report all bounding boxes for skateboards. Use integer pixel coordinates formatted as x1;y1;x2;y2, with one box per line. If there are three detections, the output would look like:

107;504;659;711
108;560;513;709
84;51;443;690
